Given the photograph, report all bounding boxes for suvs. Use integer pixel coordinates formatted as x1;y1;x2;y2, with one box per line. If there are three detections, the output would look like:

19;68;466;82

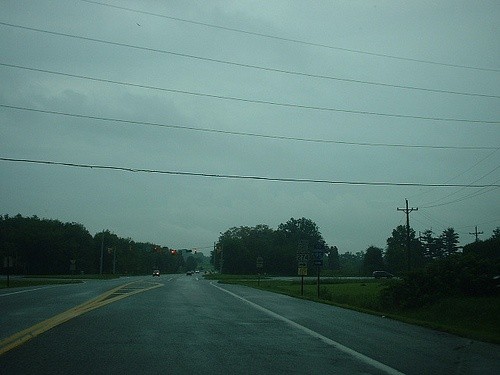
153;270;161;277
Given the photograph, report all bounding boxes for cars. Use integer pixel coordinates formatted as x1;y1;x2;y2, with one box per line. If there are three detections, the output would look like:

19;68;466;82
373;271;394;278
187;270;200;275
